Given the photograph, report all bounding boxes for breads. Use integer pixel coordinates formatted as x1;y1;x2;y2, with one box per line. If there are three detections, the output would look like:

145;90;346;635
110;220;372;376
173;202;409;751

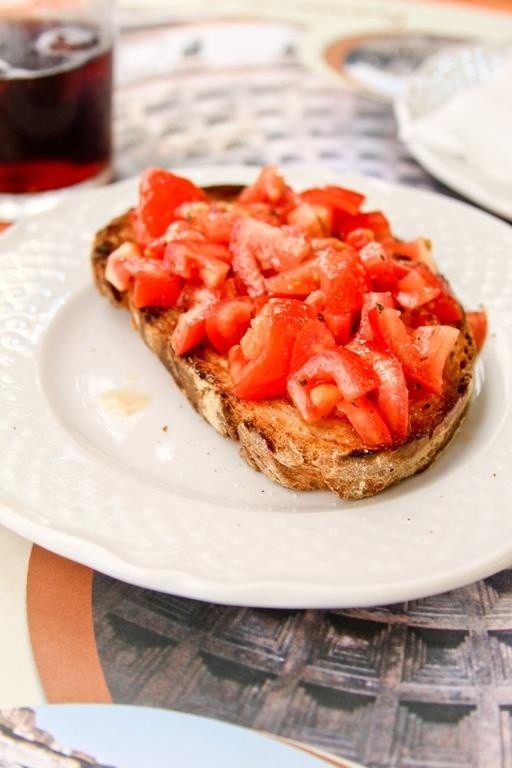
90;181;478;503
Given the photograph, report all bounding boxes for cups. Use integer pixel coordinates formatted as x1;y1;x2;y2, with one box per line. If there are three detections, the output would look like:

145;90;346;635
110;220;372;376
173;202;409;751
0;2;120;223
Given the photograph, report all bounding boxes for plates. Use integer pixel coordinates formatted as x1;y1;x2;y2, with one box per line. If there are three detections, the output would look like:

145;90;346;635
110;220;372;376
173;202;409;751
1;700;355;766
0;166;512;618
289;13;499;103
388;36;512;222
